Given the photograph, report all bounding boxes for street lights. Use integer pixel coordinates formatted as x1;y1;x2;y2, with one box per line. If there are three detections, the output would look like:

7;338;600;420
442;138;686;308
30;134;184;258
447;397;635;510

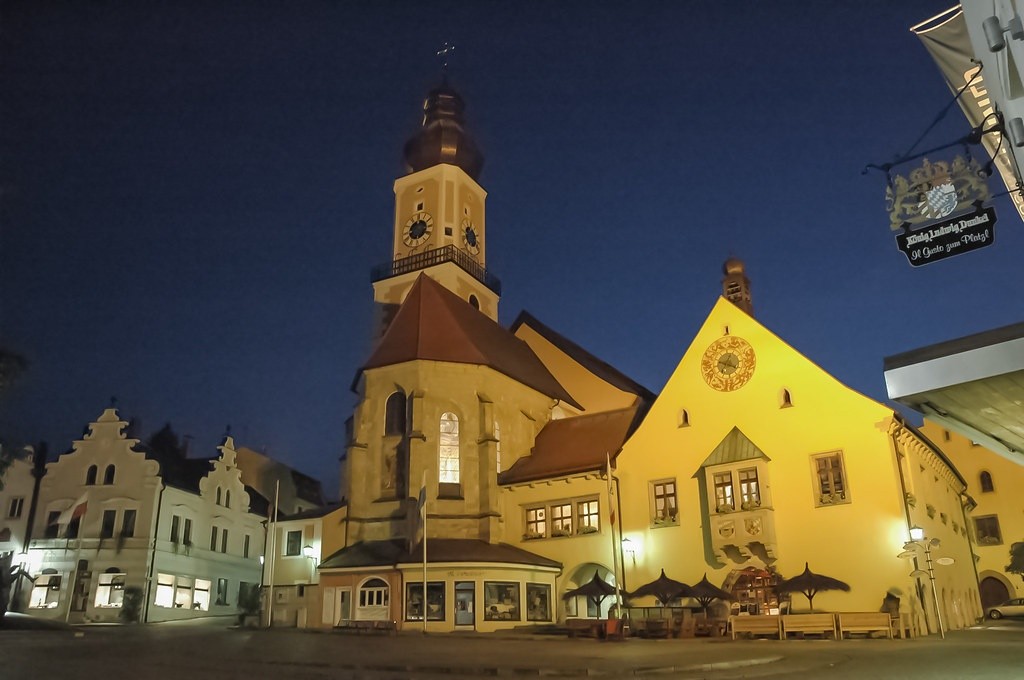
897;536;955;638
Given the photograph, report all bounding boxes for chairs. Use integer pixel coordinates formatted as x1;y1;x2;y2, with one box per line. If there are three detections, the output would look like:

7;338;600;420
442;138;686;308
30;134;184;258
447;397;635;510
600;619;624;641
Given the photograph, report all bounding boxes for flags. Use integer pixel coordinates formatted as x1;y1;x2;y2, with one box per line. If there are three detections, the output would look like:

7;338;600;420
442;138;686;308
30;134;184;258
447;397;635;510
416;473;426;543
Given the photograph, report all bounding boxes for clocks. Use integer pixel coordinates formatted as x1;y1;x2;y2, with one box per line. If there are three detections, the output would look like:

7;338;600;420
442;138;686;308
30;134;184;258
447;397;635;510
402;212;434;247
701;336;756;392
461;218;480;255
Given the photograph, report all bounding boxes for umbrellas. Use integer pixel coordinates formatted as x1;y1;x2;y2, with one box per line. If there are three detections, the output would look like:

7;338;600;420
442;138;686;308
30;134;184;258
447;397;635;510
771;562;851;611
562;568;732;624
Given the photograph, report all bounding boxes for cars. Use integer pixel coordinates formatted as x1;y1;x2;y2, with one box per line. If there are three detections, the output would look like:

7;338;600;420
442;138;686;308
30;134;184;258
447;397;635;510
986;597;1024;619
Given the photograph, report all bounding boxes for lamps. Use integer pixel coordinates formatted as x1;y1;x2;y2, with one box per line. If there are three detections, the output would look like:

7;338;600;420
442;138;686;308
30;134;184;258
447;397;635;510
303;544;316;571
904;524;923;544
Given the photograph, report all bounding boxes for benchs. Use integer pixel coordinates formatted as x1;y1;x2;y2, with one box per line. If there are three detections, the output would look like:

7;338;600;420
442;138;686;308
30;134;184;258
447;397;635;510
332;619;396;635
566;619;591;641
730;613;920;639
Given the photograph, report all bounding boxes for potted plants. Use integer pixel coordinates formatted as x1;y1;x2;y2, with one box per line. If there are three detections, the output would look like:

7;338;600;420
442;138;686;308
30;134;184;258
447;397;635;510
235;583;263;629
118;587;143;626
